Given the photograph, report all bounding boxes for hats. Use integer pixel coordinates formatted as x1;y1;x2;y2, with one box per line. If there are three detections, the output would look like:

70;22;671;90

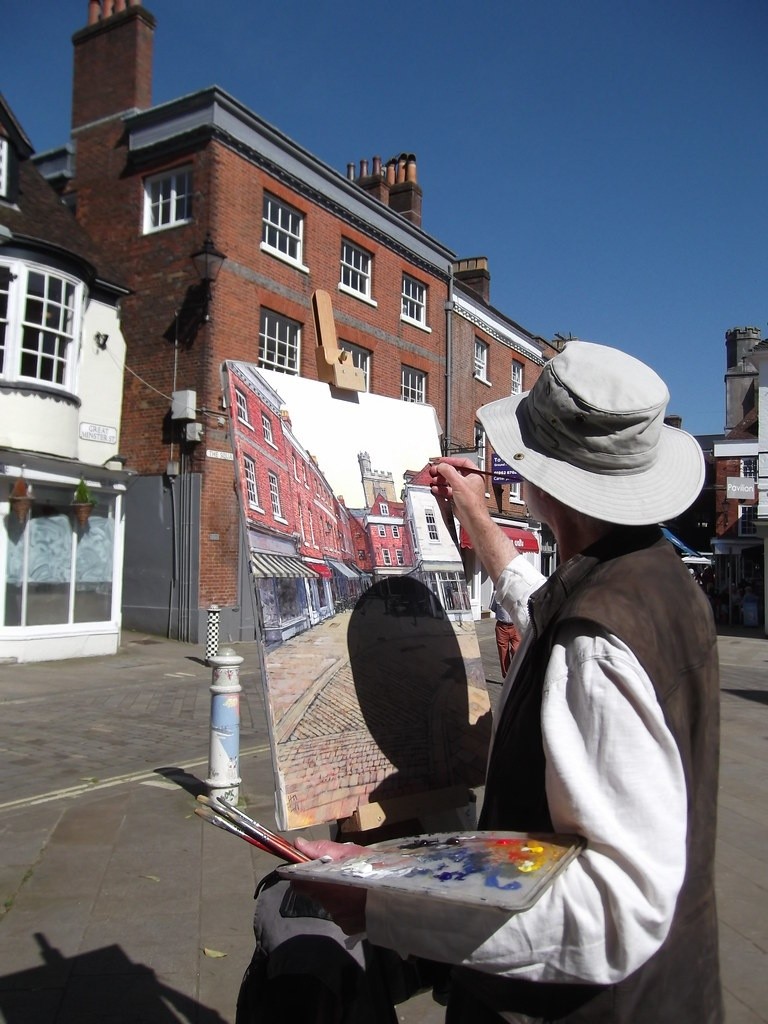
475;340;705;525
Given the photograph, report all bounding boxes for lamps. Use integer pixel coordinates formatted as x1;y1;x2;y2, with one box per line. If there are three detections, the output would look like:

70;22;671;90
721;496;729;523
190;231;228;302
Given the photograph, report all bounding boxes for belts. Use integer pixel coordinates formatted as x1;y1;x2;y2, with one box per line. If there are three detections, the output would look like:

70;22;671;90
498;620;514;626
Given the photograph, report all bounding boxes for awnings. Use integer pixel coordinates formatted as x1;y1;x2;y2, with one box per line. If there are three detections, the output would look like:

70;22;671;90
460;522;540;554
662;527;702;559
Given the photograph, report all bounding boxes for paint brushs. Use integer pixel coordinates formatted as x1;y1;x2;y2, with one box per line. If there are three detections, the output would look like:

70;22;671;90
195;792;313;864
433;460;526;484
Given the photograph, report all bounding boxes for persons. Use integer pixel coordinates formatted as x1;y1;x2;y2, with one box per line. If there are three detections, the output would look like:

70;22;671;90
490;590;522;678
295;340;727;1024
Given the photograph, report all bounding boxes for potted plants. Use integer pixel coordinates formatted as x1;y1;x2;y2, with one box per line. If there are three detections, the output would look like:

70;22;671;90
7;475;36;526
71;477;95;530
